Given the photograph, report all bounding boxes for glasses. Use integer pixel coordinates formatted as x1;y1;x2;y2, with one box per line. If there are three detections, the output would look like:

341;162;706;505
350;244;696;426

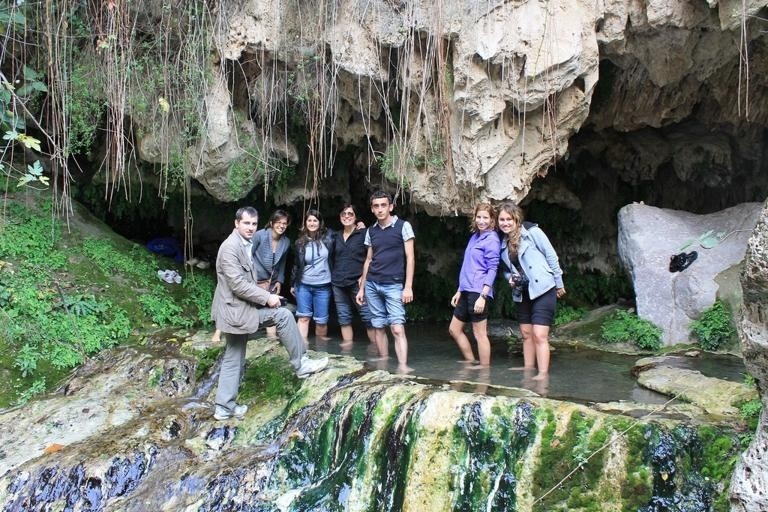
340;211;354;217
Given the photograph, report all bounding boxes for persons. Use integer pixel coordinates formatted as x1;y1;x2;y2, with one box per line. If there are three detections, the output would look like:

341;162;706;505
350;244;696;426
213;208;291;340
448;202;502;370
449;365;494;395
496;202;566;381
519;373;551;399
354;190;418;374
289;210;366;346
211;206;330;420
326;201;377;349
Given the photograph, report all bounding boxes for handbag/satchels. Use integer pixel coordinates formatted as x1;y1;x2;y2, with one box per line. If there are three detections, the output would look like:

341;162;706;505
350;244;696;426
256;279;272;291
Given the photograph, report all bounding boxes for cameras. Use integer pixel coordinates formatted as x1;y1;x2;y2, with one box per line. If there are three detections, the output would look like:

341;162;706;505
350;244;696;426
278;297;288;307
512;274;530;297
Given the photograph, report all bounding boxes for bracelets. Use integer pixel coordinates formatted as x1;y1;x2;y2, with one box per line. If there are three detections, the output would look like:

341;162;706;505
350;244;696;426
480;292;489;299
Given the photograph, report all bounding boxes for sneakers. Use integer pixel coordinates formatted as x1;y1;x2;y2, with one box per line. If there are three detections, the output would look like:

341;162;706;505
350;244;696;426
214;404;248;421
295;357;329;379
